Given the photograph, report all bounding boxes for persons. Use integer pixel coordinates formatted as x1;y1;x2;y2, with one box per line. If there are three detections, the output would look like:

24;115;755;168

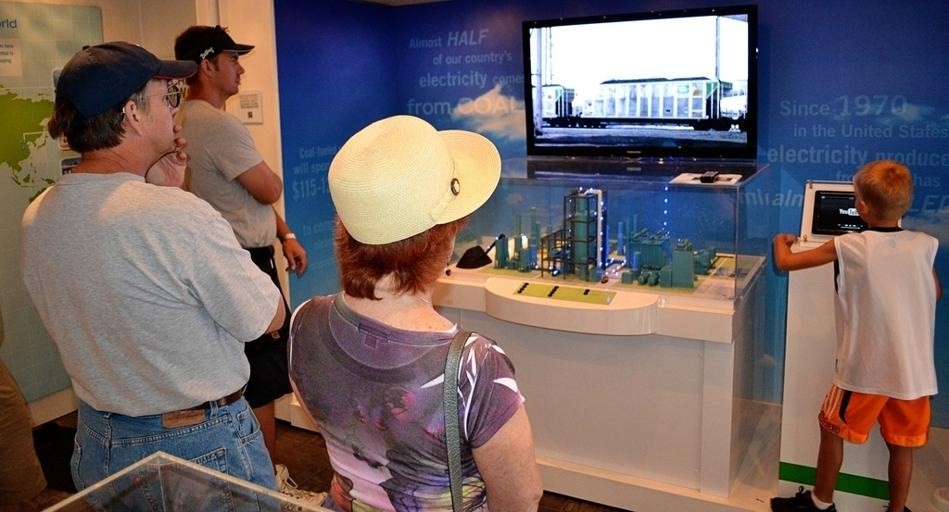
174;25;308;491
17;41;289;510
0;359;90;512
287;114;546;512
770;160;940;512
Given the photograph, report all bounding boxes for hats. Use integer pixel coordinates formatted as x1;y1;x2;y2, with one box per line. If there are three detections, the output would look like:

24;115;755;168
328;115;502;245
55;41;198;121
174;24;255;66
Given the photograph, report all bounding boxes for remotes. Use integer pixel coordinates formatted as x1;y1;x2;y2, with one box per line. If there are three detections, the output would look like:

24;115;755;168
699;170;720;183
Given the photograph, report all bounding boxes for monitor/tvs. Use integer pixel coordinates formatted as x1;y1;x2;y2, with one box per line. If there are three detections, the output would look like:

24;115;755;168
522;5;758;159
811;190;867;234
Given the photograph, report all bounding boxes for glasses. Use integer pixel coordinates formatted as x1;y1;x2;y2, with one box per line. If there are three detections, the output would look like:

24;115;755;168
119;83;183;121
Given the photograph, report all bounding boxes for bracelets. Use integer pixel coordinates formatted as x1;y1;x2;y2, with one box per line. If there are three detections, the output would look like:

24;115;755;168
281;231;296;242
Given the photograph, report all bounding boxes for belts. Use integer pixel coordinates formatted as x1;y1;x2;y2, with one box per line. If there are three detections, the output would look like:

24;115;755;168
179;384;248;412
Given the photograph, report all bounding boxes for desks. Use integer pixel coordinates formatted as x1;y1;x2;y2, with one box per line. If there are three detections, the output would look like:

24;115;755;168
40;450;333;511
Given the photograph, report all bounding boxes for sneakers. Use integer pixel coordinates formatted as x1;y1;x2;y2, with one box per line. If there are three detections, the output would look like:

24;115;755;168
770;485;836;512
274;464;329;512
